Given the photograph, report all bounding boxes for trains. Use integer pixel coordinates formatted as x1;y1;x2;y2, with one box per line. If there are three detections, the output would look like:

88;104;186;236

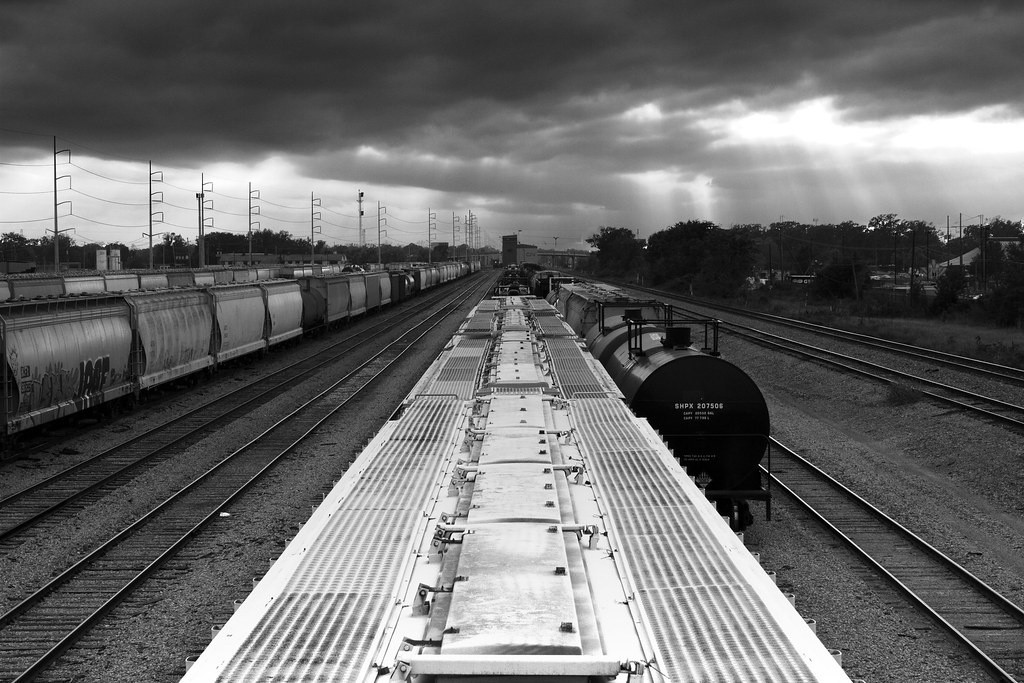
0;260;481;437
0;264;369;303
520;261;771;530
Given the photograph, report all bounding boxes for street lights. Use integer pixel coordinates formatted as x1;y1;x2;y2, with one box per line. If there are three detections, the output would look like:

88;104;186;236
553;236;559;246
196;193;204;257
